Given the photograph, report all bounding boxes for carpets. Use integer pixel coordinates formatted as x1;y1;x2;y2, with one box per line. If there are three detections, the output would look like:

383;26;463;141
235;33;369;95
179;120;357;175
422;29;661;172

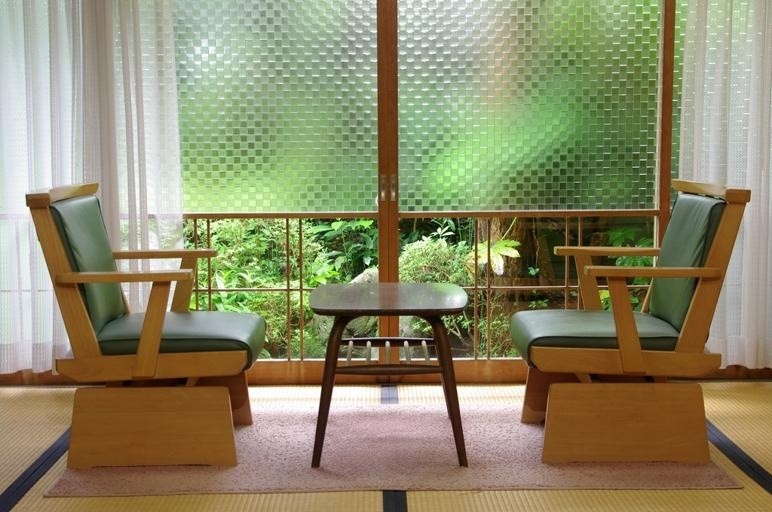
42;405;746;497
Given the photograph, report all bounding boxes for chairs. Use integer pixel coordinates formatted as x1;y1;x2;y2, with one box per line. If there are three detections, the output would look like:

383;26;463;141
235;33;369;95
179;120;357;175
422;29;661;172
24;184;267;468
509;178;751;466
